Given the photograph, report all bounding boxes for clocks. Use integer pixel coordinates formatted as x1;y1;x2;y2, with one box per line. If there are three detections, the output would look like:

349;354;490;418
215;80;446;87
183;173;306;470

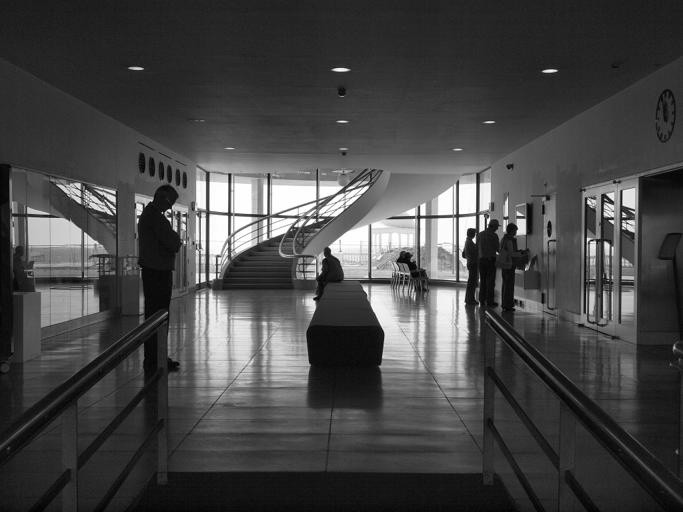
654;89;676;143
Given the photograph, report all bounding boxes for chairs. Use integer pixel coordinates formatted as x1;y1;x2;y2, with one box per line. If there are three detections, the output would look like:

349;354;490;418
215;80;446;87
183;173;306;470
389;261;429;296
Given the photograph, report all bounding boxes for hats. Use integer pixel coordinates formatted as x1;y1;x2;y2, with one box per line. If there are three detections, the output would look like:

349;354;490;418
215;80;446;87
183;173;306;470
489;219;501;226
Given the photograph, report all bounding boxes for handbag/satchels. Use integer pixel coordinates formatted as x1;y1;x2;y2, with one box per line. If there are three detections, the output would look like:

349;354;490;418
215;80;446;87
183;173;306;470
496;249;513;270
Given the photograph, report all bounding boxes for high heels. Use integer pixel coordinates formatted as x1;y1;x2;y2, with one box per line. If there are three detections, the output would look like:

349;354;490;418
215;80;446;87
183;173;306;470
501;305;516;311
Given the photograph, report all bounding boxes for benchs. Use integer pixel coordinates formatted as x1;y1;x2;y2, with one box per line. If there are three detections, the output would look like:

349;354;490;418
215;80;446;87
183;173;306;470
306;281;384;367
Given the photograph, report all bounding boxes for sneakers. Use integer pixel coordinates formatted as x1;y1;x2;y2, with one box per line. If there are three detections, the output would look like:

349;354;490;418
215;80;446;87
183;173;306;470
143;358;180;371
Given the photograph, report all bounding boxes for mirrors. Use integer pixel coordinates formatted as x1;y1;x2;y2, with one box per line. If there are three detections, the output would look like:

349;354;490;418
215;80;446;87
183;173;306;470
502;191;509;233
515;203;528;236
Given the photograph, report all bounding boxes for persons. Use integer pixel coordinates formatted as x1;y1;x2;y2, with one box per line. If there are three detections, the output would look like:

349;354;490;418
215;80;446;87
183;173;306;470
10;246;25;291
395;250;428;292
475;218;501;307
137;183;182;368
463;304;519;391
312;246;344;301
401;251;430;292
495;222;529;312
460;227;479;306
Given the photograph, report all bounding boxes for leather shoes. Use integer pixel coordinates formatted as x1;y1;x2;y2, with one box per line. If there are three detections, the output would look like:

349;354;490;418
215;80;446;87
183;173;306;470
465;299;498;306
